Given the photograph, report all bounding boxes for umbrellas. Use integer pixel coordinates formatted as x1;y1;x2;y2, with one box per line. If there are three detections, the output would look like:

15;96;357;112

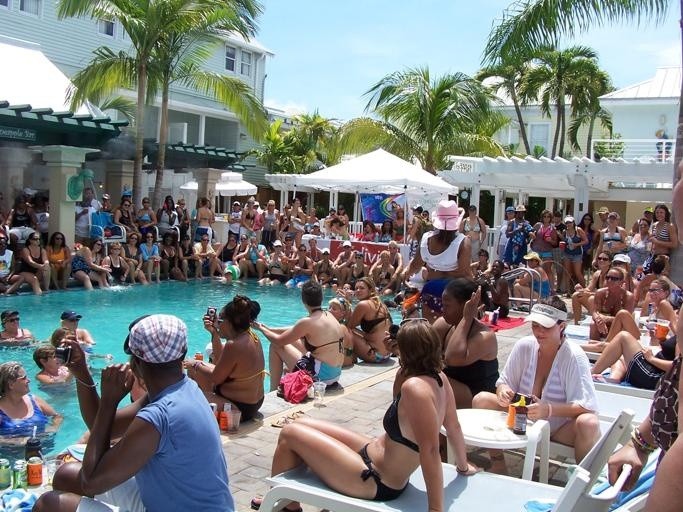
266;147;459;222
180;170;258;214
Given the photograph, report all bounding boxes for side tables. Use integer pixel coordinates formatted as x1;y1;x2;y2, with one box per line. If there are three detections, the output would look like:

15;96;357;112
0;467;77;512
440;409;550;485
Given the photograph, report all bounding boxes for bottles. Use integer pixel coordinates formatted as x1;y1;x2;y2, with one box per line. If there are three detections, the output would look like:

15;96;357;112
12;465;27;490
27;457;43;485
220;411;230;432
514;395;528;435
375;232;379;243
15;460;29;467
24;438;42;462
567;237;574;252
0;458;11;490
224;402;231;412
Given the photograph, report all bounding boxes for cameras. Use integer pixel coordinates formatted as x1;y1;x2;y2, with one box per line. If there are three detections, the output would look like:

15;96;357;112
206;307;216;323
512;392;531;406
55;344;72;368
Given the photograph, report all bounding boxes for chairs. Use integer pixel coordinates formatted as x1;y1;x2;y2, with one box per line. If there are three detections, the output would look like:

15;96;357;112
577;311;649;324
348;222;364;237
373;224;383;230
562;325;605;339
88;212;126;257
485;225;501;264
590;384;655;423
4;224;28;245
569;340;662;360
257;410;659;512
154;224;180;242
494;421;640;483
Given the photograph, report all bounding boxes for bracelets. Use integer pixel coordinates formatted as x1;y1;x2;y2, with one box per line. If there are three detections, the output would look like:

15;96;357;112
547;402;552;420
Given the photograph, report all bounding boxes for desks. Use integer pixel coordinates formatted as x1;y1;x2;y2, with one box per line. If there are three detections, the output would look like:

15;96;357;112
300;237;410;272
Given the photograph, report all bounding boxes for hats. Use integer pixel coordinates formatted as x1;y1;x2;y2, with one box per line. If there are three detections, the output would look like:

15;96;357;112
505;207;515;211
565;216;574;223
611;254;631;264
411;205;423;213
1;310;20;324
355;253;362;258
608;212;620;221
342;240;351;247
273;240;282;247
595;207;609;215
234;201;240;205
515;205;526;212
524;252;542;265
123;314;188;364
321;248;330;255
523;304;567;328
61;311;81;321
431;200;466;231
285;235;292;241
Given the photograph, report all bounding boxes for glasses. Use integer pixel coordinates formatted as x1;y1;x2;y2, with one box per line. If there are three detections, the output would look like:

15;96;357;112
6;318;20;323
54;236;64;240
202;239;209;242
184;237;190;240
354;256;362;260
286;239;292;241
30;237;42;241
299;249;306;252
343;245;351;248
143;201;150;204
234;204;239;207
543;215;551;218
146;235;154;238
647;288;663;293
130;236;138;240
45;354;56;360
12;376;26;382
124;203;131;207
95;242;103;246
605;275;621;282
338;297;346;308
598;256;609;261
274;245;282;247
400;317;429;326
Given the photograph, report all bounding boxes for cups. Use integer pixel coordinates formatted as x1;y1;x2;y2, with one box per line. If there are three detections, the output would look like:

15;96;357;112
226;408;241;436
313;382;325;406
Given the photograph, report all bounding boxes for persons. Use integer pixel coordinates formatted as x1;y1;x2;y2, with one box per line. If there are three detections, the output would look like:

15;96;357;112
561;215;586;298
36;197;50;248
529;211;558;294
429;281;500;409
581;279;677;347
253;201;264;244
0;217;7;238
256;241;288;289
479;259;512;321
325;297;358;370
285;234;299;258
513;252;551;312
177;199;190;240
648;203;678;258
177;235;202;281
261;201;280;253
51;326;93;364
309;223;323;237
102;242;130;286
329;217;351;242
496;208;519;258
346;252;370;290
113;190;139;228
0;361;63;449
71;240;113;290
307;238;322;262
182;295;264;424
190;198;201;243
607;354;683;491
34;344;72;386
136;197;158;241
24;188;37;208
337;203;350;226
504;206;535;268
285;198;308;248
159;233;189;284
592;211;628;268
408;205;423;263
214;235;240;275
460;205;487;261
331;241;355;287
625;219;646;249
362;222;378;242
423;209;430;227
191;197;212;245
163;195;173;210
476;295;604;476
306;208;319;224
232;233;249;267
222;264;241;284
18;232;50;295
391;199;401;212
589;306;683;391
256;281;346;390
120;234;149;286
60;311;95;348
632;218;655;254
578;214;593;274
323;207;338;234
51;328;71;364
400;200;474;323
471;250;490;278
75;188;103;248
240;237;269;281
157;198;181;245
311;252;334;289
598;204;608;221
283;244;314;291
553;212;566;294
251;316;484;512
393;208;406;242
6;195;44;260
32;313;235;511
645;432;683;512
635;252;680;307
193;233;218;278
379;221;394;242
385;260;428;311
574;254;611;325
139;233;162;284
503;261;511;274
243;195;262;214
113;200;142;244
45;232;71;292
0;309;35;348
644;206;653;223
240;199;256;243
372;252;397;295
387;241;402;274
71;243;83;255
102;193;116;213
340;276;394;366
207;200;216;242
587;267;634;341
0;237;25;297
227;201;243;244
279;204;290;243
612;254;632;273
358;219;368;241
